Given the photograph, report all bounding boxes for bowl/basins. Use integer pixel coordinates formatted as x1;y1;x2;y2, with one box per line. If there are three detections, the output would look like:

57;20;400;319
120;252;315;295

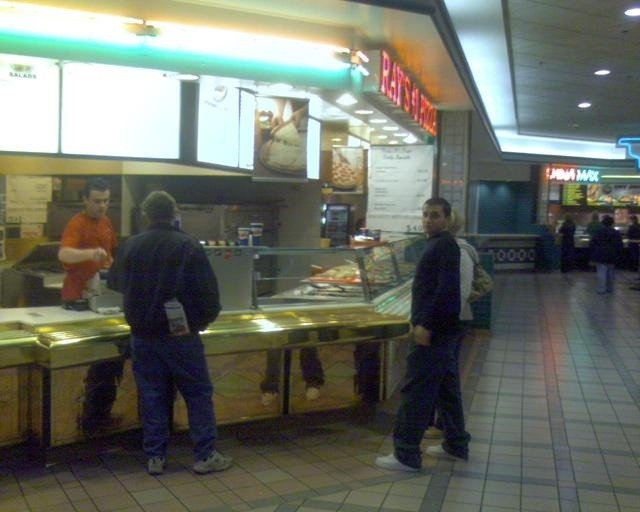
199;240;236;246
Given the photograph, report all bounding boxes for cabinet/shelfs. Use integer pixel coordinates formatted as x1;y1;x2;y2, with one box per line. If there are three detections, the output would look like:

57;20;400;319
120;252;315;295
254;235;425;402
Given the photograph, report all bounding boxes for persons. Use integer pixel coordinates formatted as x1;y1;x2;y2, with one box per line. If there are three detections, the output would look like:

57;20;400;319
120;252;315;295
261;349;325;405
108;191;232;475
586;212;600;234
421;208;478;438
558;214;576;272
58;178;117;301
590;215;623;294
627;215;640;270
375;198;470;472
77;358;124;433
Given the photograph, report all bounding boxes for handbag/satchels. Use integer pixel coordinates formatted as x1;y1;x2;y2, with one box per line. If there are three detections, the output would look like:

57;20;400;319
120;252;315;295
466;262;496;304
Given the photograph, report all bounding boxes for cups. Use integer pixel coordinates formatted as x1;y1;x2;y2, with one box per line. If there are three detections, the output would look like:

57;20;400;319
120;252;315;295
319;238;332;248
371;229;381;241
361;227;369;237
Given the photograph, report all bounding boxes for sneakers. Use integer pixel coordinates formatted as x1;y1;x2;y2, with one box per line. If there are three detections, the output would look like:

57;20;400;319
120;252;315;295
425;443;469;462
192;448;235;475
375;452;422;473
147;455;169;476
